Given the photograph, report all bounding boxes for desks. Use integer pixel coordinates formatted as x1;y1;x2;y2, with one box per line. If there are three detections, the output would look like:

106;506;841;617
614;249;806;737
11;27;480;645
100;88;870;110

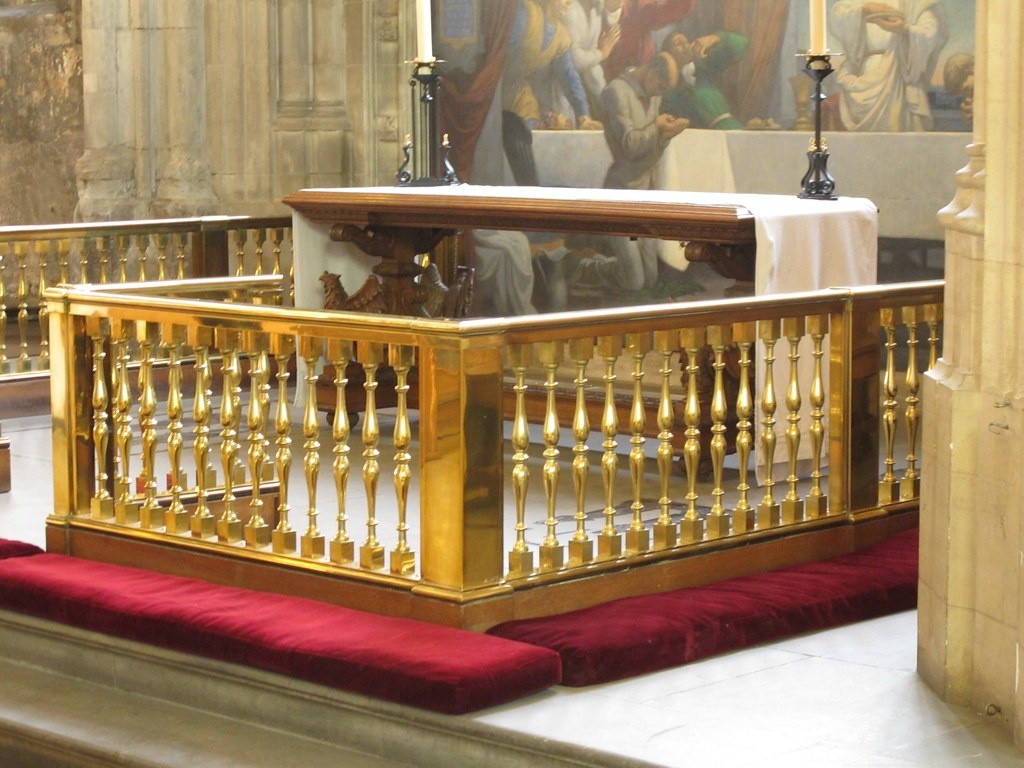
284;174;877;472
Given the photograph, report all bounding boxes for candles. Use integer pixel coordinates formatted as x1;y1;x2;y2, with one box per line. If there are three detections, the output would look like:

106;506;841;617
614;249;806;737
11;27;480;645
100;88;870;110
416;0;432;59
809;0;828;53
